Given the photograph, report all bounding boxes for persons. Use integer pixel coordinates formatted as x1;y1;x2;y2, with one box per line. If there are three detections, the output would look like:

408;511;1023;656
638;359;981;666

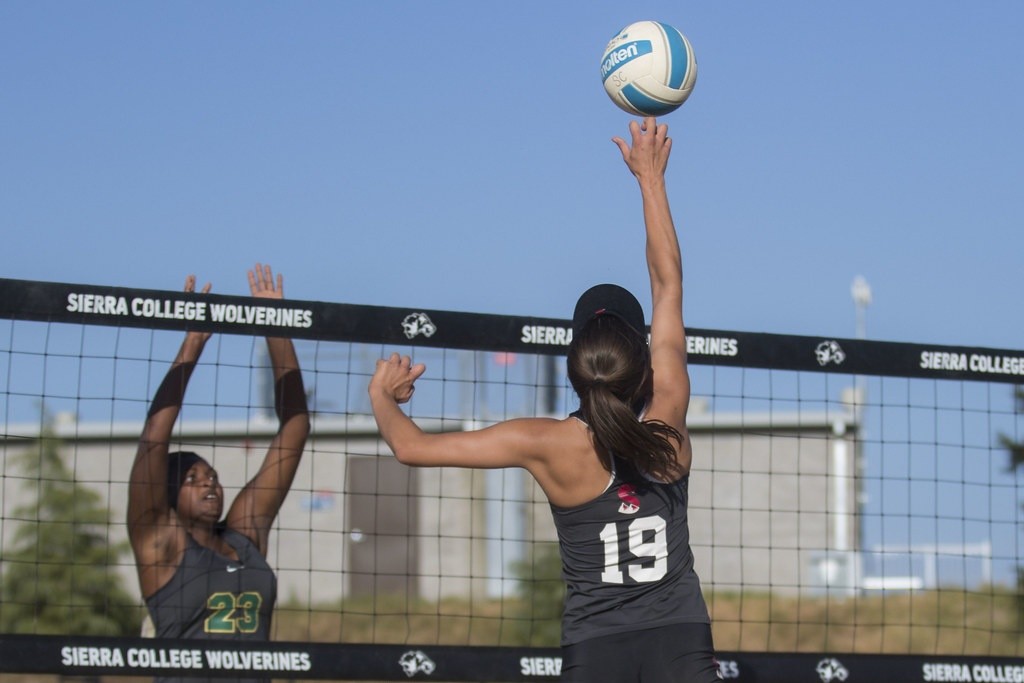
368;115;717;682
126;262;310;683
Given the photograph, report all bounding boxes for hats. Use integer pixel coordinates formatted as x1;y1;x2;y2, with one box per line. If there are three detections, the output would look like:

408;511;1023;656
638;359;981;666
574;284;648;341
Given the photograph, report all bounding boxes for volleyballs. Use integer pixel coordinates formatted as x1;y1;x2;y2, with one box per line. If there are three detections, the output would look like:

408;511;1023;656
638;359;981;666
600;20;698;116
